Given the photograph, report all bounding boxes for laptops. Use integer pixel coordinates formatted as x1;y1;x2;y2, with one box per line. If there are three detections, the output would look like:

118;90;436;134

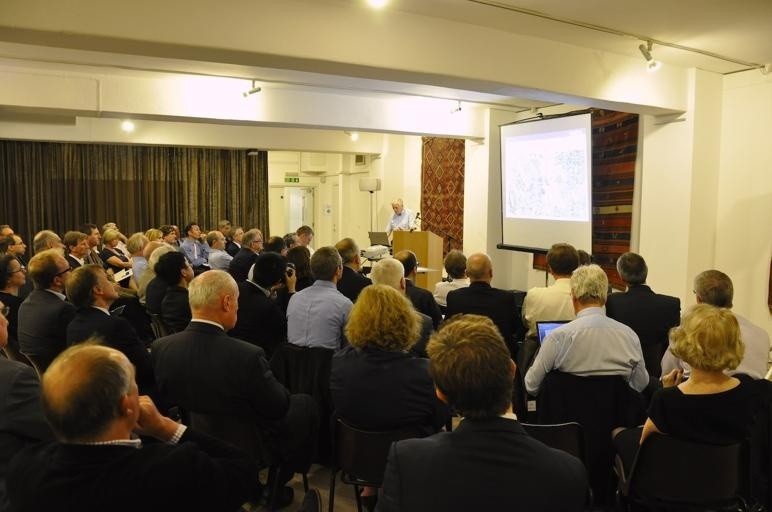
368;231;392;248
536;320;575;346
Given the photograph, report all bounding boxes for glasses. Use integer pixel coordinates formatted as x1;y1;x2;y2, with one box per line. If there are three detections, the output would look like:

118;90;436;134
54;265;76;280
12;242;23;246
1;262;26;279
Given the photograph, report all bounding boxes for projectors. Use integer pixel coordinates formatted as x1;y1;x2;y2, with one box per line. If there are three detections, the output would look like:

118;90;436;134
361;244;389;259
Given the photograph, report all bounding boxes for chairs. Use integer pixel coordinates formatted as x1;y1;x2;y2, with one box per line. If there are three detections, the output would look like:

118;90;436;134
1;262;772;512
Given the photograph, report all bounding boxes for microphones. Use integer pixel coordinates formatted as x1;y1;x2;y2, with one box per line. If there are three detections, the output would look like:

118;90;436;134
415;212;419;219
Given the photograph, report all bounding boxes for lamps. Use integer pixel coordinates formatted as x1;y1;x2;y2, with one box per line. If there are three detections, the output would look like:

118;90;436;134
241;78;263;98
638;38;664;75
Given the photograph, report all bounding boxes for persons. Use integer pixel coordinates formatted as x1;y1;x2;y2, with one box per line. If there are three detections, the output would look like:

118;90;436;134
374;314;594;512
641;298;771;502
385;197;418;237
12;333;217;512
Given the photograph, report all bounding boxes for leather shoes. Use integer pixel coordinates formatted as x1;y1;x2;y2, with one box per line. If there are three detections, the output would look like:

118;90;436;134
298;486;326;512
357;487;378;506
270;485;295;510
